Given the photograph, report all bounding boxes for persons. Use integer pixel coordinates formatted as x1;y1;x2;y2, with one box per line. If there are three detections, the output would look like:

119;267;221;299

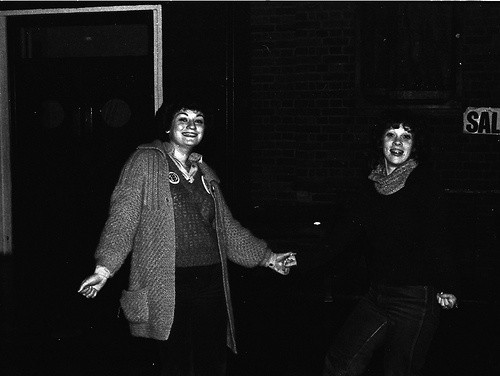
322;116;463;376
77;96;297;374
